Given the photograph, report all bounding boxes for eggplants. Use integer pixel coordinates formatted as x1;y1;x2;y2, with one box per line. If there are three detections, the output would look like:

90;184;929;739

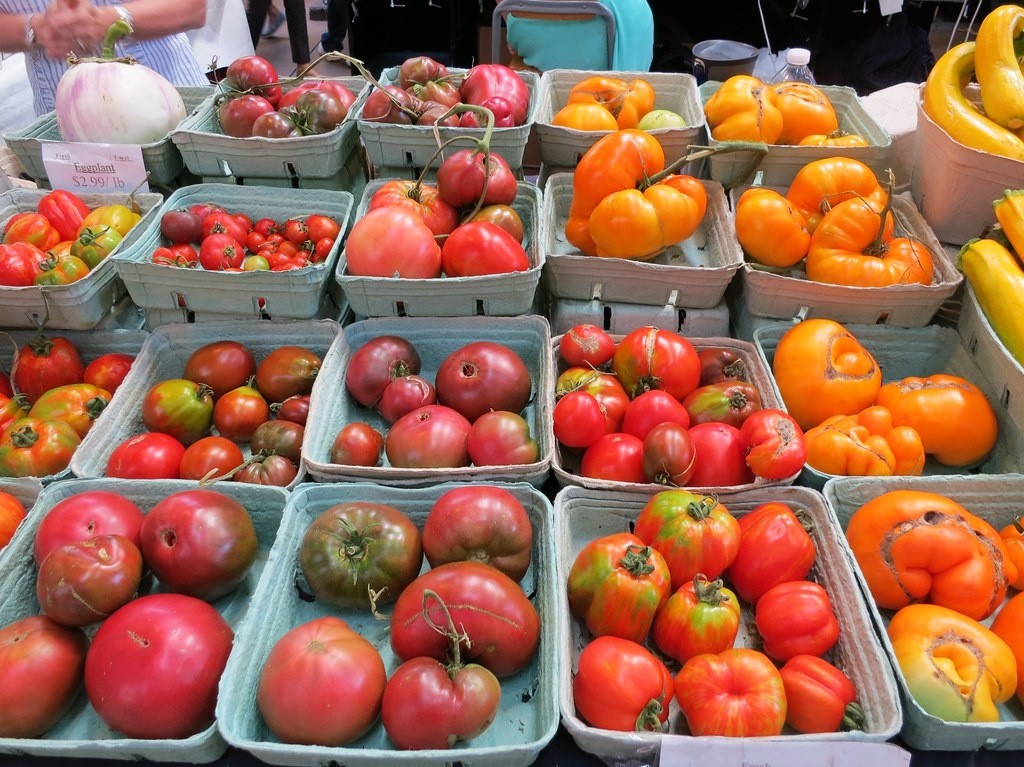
57;21;187;147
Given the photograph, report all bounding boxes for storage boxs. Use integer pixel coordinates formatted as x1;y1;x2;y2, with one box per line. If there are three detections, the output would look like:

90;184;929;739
0;67;1024;767
477;21;510;66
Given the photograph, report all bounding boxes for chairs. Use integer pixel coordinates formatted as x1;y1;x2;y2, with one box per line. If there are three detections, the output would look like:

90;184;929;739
490;0;616;70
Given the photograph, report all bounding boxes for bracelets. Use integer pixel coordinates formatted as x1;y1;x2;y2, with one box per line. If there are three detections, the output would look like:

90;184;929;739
114;6;133;29
24;14;34;49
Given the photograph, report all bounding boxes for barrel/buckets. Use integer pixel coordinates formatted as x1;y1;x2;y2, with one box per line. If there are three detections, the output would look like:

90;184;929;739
692;39;759;82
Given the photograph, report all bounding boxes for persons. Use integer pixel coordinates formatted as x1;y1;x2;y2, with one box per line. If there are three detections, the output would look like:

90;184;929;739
260;4;285;38
0;0;207;119
318;0;354;61
245;0;325;79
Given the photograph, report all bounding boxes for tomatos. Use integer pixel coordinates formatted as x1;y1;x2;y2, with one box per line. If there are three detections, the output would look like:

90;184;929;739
0;486;1024;750
0;128;935;307
217;56;870;148
0;315;996;490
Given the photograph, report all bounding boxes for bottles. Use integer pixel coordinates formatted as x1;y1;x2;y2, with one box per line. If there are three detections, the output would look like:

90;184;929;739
772;48;817;86
